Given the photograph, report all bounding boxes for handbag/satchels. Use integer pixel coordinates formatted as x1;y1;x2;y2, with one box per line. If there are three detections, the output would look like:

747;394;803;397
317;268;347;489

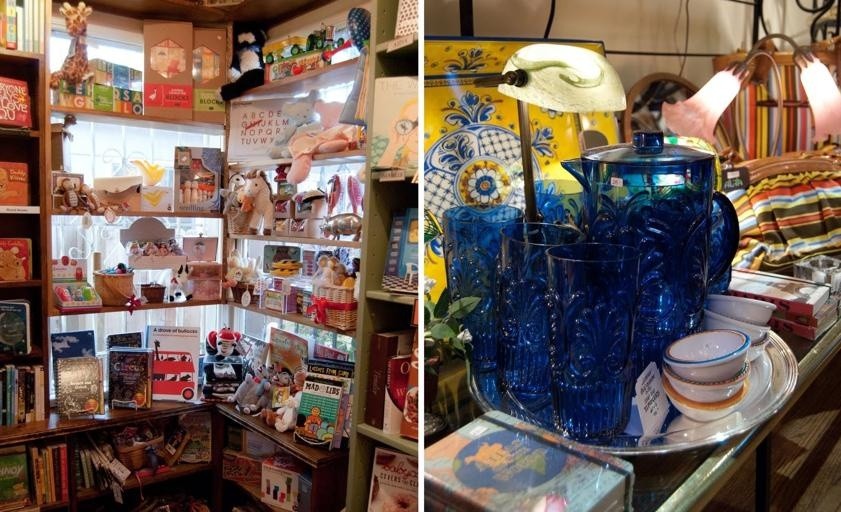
727;152;841;272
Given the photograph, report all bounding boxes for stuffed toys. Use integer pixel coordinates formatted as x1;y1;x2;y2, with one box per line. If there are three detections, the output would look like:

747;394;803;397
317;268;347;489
48;2;373;512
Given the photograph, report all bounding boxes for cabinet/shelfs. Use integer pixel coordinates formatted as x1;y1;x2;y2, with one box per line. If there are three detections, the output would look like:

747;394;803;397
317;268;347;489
217;0;347;511
0;0;50;443
50;0;236;512
346;0;419;511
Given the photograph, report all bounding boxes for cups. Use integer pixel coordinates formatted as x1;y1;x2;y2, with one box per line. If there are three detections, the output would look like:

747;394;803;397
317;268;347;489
440;130;632;446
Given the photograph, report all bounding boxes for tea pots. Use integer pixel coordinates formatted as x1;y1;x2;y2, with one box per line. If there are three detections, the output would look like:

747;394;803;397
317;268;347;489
561;124;739;419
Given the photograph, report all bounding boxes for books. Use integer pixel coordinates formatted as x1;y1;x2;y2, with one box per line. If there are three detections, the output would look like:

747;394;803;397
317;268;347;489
0;0;51;512
366;75;417;512
423;411;634;512
725;266;838;341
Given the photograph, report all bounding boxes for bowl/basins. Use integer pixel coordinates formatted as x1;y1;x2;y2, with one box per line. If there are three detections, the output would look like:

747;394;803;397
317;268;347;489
664;289;778;422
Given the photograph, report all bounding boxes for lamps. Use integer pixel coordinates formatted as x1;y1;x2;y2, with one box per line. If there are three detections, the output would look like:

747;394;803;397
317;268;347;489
473;42;628;221
662;33;841;156
